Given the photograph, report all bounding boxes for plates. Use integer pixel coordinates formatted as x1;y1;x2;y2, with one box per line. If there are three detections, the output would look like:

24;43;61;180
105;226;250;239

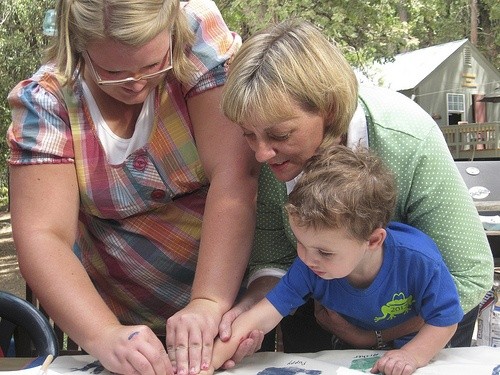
469;186;489;199
466;167;480;175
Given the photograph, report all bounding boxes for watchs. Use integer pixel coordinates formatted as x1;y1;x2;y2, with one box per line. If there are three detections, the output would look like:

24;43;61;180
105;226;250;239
375;328;388;349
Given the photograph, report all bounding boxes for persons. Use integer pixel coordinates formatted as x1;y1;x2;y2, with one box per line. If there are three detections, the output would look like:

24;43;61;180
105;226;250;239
187;138;463;375
221;17;495;354
6;0;258;375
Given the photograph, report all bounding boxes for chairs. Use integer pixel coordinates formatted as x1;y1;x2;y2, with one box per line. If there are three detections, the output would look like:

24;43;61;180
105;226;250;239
0;291;59;357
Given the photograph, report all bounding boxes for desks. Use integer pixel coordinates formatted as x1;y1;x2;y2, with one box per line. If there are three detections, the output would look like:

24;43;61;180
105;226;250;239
0;346;500;375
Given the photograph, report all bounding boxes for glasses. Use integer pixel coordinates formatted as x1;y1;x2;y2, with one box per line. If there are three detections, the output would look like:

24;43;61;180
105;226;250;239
77;31;172;85
